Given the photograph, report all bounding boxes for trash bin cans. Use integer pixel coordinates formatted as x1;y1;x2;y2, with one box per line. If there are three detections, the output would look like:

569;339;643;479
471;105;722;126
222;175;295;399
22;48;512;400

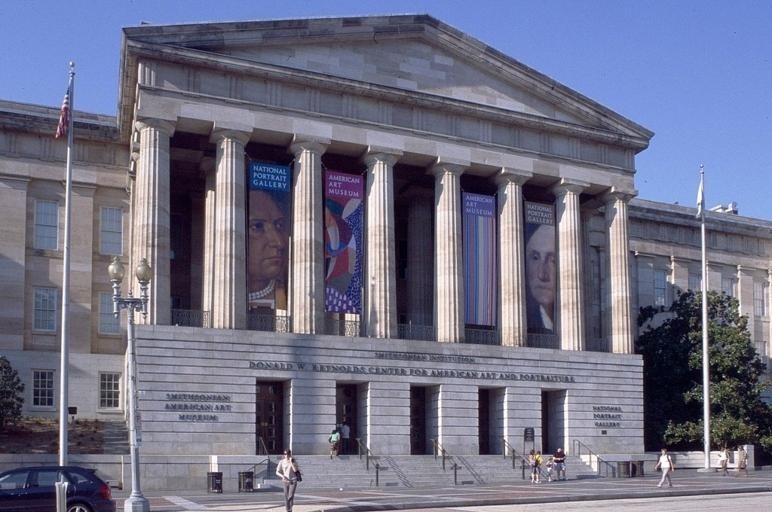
617;461;630;478
207;472;223;493
238;471;253;492
632;461;644;477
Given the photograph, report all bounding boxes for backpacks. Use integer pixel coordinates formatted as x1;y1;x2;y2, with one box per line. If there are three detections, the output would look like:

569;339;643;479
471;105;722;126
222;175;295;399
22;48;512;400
531;455;540;468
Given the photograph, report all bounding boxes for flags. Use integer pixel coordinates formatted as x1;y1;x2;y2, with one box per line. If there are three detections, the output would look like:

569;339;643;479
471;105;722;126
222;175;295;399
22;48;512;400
55;86;70;139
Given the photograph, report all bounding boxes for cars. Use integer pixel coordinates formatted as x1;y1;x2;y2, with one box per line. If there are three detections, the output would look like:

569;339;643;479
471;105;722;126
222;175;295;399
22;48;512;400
0;466;116;512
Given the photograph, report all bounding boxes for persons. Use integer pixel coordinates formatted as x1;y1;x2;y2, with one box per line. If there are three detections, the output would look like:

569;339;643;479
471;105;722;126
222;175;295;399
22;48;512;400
655;448;675;488
735;446;749;479
524;224;557;333
529;449;542;484
275;448;298;512
248;188;288;314
330;421;350;460
553;448;568;481
546;457;554;482
718;446;730;476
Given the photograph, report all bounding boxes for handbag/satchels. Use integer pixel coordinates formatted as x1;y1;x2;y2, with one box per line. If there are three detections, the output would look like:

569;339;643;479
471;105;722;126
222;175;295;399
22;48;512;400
290;458;302;482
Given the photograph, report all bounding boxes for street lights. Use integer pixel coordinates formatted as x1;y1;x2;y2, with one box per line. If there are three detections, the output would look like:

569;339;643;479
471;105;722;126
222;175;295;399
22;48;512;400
107;256;151;512
